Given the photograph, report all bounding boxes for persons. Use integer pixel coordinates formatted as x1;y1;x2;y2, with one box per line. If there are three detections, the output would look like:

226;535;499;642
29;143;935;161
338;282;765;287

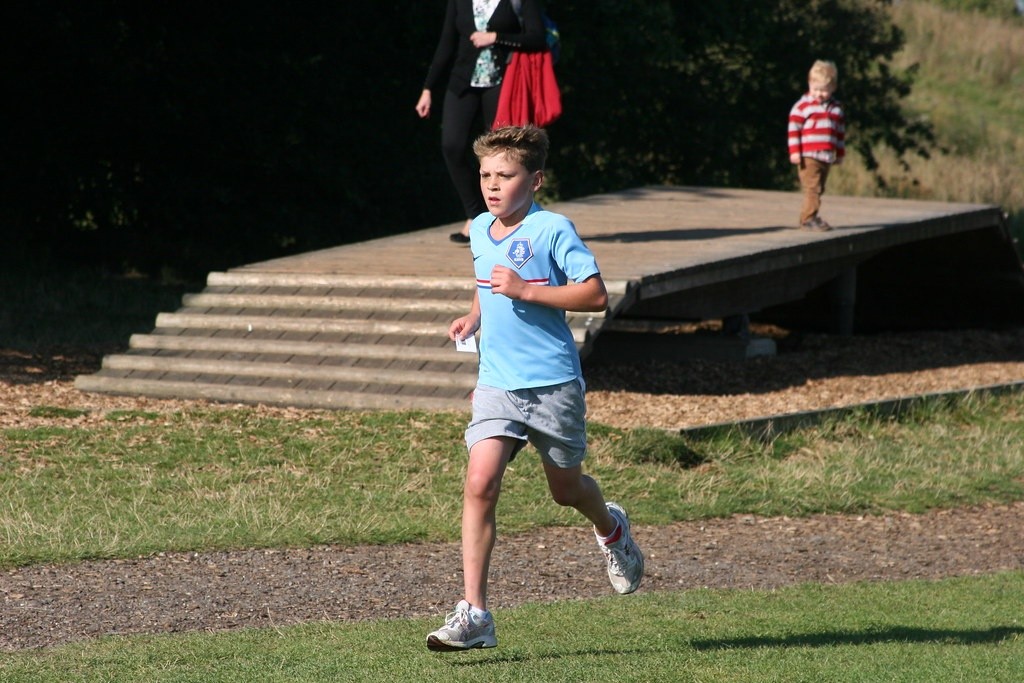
787;59;847;234
413;0;561;245
420;122;644;655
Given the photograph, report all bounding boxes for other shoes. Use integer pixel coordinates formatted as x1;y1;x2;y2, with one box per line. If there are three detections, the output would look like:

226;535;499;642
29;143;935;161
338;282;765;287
802;217;833;232
449;232;471;247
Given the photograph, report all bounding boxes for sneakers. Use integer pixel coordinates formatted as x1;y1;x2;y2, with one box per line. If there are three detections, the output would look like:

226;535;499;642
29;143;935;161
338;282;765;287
593;502;644;594
427;600;498;652
